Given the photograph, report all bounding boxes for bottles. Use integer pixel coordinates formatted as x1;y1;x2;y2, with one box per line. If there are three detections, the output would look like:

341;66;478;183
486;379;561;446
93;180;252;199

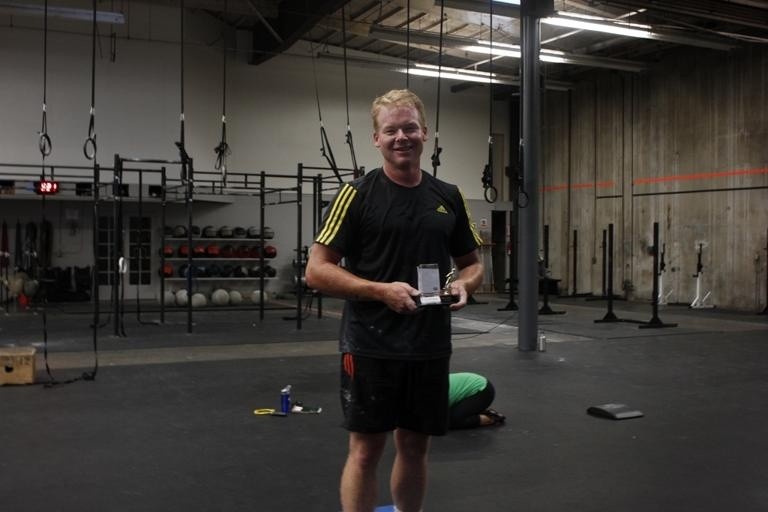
280;384;290;412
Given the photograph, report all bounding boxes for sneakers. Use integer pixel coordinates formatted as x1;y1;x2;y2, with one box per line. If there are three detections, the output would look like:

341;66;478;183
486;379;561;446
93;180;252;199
488;409;507;424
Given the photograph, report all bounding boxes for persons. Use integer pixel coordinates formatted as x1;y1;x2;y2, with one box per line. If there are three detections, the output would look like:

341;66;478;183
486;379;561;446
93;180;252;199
305;90;484;512
448;372;506;428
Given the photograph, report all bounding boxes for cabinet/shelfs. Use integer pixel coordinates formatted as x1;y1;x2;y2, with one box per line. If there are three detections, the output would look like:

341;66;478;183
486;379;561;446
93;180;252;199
160;236;280;282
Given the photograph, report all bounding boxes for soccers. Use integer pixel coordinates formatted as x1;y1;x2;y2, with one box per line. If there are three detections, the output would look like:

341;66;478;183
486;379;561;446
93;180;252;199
156;226;276;307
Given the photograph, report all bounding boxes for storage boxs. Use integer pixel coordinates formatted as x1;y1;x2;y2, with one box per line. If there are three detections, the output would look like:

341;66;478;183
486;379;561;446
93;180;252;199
0;346;36;384
